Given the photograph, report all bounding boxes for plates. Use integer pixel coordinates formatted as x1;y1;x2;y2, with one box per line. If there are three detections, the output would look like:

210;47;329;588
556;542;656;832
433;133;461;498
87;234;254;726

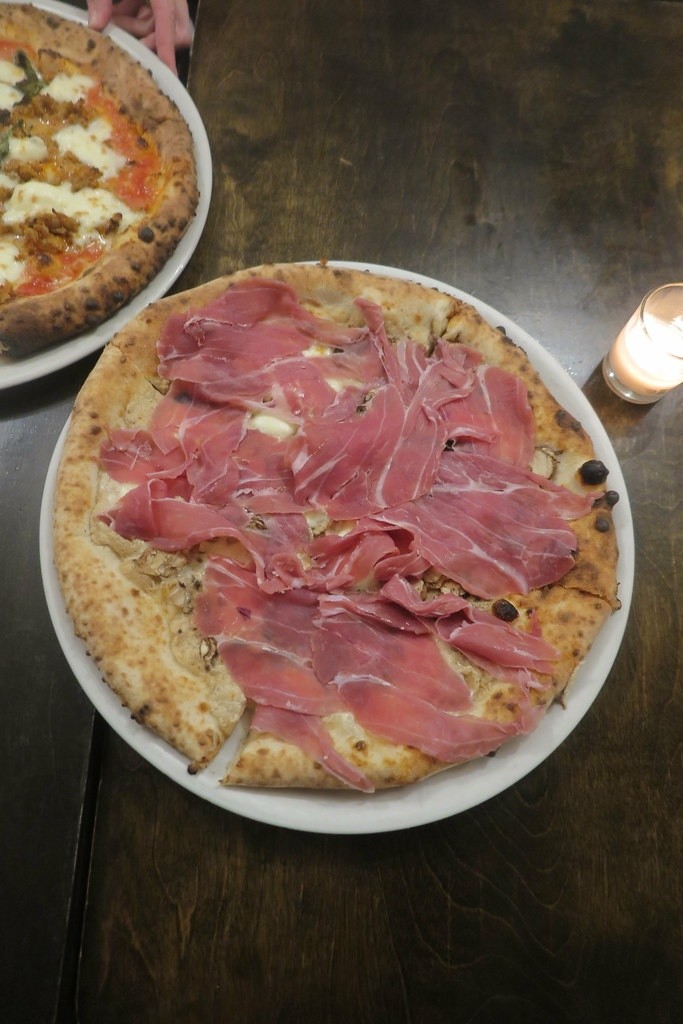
40;261;631;834
0;0;213;388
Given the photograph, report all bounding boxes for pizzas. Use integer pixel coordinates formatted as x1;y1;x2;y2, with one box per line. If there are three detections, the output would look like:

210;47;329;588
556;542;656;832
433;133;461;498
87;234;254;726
51;265;620;795
0;2;201;358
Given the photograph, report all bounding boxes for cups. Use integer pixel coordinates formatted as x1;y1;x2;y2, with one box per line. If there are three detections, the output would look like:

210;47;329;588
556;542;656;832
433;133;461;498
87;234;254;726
602;283;683;404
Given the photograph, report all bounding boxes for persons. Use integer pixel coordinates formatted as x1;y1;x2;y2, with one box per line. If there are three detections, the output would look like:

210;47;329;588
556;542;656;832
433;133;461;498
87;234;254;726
86;0;195;74
